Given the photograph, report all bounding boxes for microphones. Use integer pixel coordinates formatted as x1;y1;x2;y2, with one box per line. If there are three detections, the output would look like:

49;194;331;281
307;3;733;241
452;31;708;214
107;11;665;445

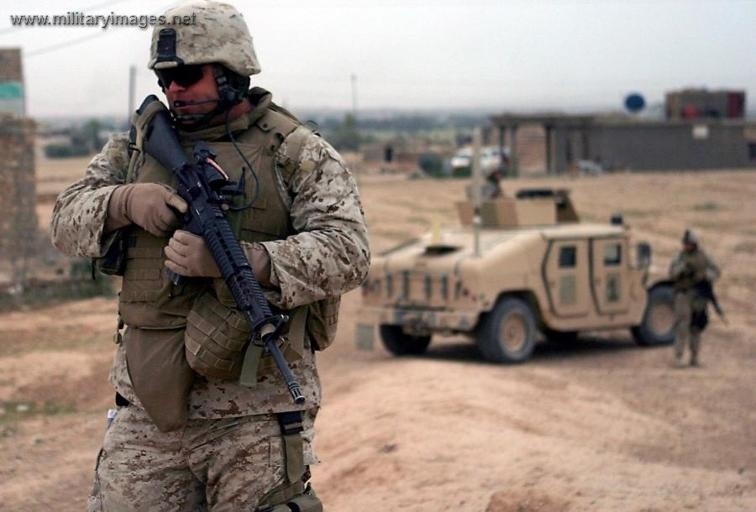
174;98;220;108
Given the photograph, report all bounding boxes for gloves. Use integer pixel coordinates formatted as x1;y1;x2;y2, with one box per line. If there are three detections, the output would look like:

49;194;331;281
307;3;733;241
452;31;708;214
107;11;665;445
164;230;223;278
107;183;188;236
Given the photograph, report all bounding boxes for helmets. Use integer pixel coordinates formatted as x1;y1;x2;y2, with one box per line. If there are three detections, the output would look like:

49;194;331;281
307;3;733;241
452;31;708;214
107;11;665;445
684;231;698;245
149;0;262;78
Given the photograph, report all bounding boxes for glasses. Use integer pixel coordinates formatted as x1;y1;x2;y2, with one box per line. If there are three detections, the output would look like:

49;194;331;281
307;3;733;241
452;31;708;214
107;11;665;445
154;65;205;88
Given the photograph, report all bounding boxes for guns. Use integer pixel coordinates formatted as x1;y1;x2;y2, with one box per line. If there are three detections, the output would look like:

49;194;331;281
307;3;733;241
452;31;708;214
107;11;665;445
686;264;728;327
128;94;306;407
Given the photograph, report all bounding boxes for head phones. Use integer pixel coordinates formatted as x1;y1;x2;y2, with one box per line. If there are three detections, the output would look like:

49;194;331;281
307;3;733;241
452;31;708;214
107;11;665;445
215;63;250;106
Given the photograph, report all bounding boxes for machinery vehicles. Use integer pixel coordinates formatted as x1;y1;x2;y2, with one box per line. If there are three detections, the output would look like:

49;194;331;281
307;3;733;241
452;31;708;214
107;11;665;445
357;187;688;365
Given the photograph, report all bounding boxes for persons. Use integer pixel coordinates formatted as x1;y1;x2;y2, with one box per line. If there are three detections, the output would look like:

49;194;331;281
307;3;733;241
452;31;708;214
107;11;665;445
50;1;372;511
481;160;507;206
669;229;721;367
